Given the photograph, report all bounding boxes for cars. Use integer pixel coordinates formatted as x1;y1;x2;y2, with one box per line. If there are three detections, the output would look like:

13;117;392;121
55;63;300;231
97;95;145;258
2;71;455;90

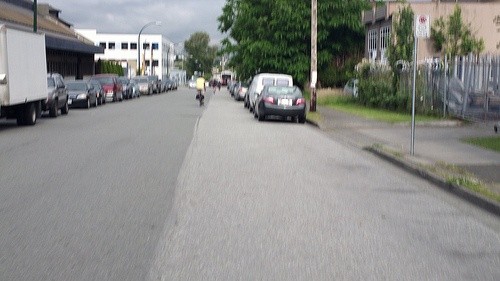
227;73;305;123
42;72;177;118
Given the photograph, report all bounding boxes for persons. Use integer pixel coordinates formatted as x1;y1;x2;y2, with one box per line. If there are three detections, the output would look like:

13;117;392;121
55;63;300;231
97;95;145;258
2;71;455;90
195;75;206;100
210;79;221;94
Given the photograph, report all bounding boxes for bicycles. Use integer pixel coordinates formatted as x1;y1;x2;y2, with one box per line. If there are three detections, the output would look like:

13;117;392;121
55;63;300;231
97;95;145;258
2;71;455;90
196;88;203;106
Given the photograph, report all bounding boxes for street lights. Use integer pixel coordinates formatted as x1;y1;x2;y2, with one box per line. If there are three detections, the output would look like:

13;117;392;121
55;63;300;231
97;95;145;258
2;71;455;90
138;21;161;76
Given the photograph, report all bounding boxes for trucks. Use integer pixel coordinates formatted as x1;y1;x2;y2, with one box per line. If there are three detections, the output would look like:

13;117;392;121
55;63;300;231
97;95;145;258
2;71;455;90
0;26;49;126
